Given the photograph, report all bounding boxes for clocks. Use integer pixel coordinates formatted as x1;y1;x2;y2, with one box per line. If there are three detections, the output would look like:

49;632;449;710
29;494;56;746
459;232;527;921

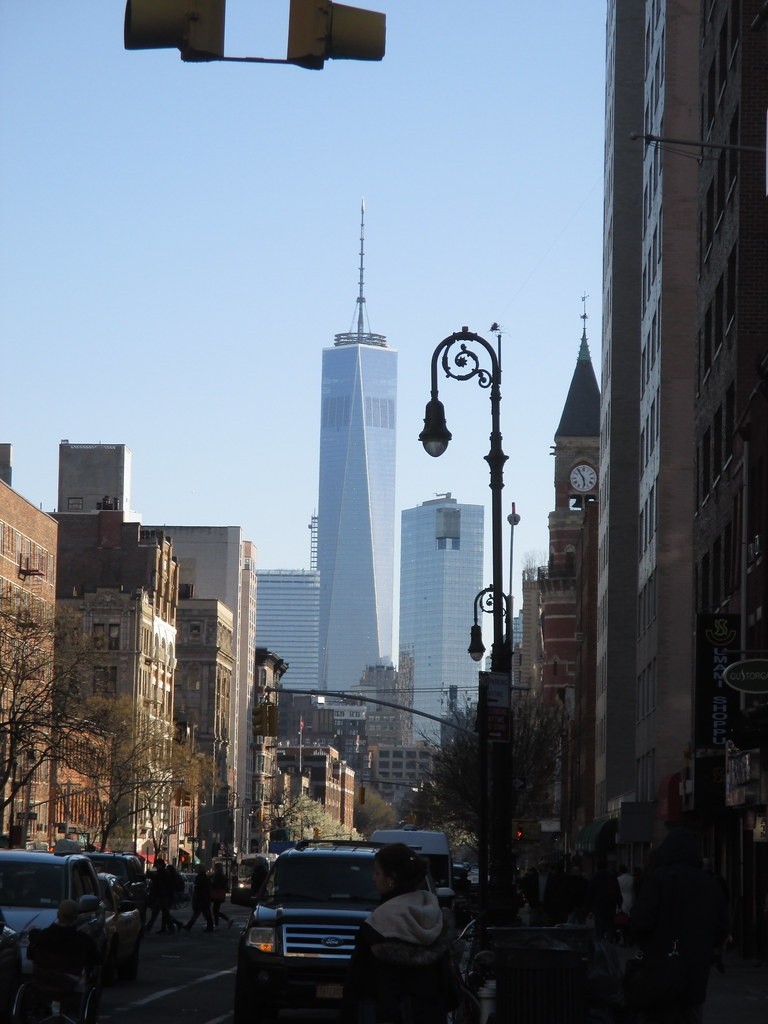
570;464;598;491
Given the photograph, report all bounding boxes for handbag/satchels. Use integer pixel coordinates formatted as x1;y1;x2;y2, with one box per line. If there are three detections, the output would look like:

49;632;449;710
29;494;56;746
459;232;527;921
209;889;225;902
585;912;596;928
613;911;633;928
623;959;690;1010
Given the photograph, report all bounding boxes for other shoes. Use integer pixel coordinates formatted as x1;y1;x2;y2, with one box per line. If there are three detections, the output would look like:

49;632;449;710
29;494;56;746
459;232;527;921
144;926;150;932
228;920;234;929
177;922;183;931
204;929;213;932
213;924;219;928
156;929;167;934
167;919;178;929
182;926;190;931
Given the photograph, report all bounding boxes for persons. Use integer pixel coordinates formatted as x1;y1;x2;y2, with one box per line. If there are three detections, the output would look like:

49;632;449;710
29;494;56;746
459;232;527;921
339;843;463;1024
143;857;268;934
517;863;644;943
631;828;729;1024
23;869;62;908
0;899;105;1024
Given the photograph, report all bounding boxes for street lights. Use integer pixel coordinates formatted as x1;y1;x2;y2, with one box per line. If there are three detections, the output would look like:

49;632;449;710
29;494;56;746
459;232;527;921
410;330;510;927
466;583;514;739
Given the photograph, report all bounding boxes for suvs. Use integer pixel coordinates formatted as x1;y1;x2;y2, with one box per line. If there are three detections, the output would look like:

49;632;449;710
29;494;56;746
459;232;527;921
234;836;458;1024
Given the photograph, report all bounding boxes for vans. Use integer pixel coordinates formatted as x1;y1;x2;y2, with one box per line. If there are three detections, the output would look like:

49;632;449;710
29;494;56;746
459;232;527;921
350;823;465;910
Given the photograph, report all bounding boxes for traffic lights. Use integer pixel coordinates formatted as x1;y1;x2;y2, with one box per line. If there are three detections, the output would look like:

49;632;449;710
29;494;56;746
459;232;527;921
179;854;188;864
181;789;190;807
251;703;267;737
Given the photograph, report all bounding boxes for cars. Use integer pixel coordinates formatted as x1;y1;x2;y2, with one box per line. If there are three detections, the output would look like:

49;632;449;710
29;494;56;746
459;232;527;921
0;839;280;1024
454;858;492;891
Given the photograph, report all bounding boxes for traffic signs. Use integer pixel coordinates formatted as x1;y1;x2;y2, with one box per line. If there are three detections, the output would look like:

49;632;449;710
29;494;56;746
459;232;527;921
16;813;37;819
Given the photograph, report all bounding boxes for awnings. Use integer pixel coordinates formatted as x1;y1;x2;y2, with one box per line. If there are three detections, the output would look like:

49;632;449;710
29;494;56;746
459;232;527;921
571;816;618;852
